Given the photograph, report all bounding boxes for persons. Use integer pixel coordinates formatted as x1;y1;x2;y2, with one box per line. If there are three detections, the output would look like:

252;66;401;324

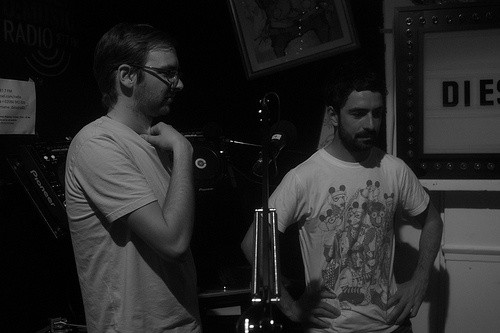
65;22;203;333
241;79;443;333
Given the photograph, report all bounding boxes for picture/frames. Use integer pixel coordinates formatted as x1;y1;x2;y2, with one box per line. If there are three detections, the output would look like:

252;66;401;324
228;0;362;81
394;0;500;179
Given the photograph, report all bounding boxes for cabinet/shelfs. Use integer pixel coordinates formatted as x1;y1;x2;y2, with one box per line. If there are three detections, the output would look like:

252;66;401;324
439;209;500;333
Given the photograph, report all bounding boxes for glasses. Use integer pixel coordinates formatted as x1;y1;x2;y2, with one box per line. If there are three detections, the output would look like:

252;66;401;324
133;65;181;88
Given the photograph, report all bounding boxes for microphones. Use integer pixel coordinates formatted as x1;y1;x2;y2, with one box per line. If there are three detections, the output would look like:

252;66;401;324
252;120;298;173
